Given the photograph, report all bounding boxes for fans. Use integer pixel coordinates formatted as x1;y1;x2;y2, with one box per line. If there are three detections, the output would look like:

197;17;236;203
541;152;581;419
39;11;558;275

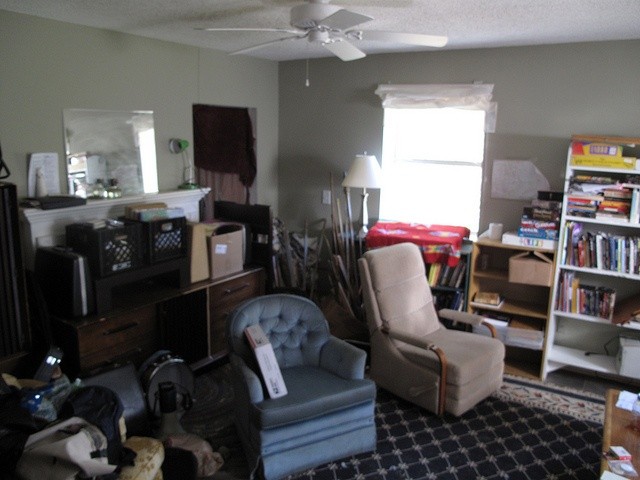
194;0;448;61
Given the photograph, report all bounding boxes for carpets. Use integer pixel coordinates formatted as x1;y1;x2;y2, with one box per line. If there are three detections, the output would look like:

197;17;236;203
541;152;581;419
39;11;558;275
163;340;606;480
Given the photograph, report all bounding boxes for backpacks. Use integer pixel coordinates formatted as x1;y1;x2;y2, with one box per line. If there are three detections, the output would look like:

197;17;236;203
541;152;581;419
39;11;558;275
57;385;137;480
15;417;118;480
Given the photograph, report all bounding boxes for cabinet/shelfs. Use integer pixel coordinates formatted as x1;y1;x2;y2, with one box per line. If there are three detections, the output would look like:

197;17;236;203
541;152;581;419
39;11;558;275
467;231;559;382
208;270;266;357
56;306;155;371
356;234;472;332
539;136;640;385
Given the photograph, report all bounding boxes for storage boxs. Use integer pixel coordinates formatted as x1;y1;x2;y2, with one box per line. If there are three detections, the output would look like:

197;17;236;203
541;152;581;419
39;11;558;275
209;222;246;279
508;251;552;288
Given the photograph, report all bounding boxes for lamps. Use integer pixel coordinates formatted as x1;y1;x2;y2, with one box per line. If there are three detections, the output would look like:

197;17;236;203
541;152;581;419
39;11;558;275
168;138;199;190
340;152;386;239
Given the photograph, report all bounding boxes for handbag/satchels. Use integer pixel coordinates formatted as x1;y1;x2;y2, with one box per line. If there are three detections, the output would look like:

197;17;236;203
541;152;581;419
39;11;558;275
179;222;210;283
138;350;194;426
0;395;49;480
2;367;72;424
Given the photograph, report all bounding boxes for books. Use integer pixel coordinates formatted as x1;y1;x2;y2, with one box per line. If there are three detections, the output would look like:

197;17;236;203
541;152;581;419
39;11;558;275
501;206;560;251
556;270;616;321
81;217;129;262
433;292;464;329
125;202;183;251
424;259;467;289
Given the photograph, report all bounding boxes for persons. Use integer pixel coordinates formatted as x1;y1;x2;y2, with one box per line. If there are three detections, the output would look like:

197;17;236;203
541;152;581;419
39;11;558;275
561;221;640;276
566;174;640;226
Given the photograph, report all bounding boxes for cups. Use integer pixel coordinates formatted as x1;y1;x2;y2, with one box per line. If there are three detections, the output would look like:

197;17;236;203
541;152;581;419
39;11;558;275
489;223;503;242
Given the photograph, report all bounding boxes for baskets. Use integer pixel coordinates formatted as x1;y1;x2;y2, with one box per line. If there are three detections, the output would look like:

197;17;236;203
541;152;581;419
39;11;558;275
81;360;147;420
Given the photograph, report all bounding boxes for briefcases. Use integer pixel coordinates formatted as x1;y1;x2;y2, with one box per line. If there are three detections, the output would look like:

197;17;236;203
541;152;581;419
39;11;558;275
29;245;97;319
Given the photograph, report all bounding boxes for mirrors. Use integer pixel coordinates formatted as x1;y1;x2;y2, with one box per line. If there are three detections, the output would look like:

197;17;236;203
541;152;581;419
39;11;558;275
62;107;160;201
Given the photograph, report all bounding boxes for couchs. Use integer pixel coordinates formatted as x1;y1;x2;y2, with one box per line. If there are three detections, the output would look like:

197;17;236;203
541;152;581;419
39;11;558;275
358;243;506;416
0;374;165;480
230;294;378;480
